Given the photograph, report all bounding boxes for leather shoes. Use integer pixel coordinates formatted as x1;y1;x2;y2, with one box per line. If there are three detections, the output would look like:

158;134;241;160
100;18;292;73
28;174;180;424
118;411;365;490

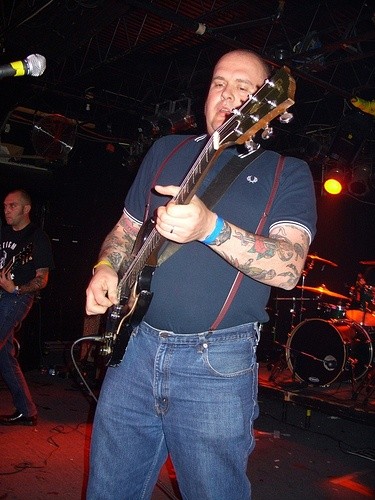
0;408;38;426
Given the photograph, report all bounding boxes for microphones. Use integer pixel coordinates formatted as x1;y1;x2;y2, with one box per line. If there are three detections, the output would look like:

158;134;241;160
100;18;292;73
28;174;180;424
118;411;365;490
0;54;47;78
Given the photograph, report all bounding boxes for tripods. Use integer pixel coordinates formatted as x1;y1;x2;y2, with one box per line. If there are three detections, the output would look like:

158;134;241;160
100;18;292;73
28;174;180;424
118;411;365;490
266;270;305;383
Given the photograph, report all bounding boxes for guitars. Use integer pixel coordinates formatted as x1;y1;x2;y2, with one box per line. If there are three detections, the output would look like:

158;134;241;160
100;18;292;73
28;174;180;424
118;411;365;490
78;66;296;366
0;241;36;279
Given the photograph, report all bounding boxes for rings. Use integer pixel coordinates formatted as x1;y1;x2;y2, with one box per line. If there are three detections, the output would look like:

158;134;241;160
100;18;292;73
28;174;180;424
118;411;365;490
170;225;174;233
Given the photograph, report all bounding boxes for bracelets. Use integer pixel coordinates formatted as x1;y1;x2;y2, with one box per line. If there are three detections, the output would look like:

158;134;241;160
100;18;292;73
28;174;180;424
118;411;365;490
13;285;19;296
92;259;114;276
200;215;224;245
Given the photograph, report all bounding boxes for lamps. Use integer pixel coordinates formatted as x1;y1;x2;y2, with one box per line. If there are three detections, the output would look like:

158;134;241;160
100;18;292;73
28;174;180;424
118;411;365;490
322;167;346;195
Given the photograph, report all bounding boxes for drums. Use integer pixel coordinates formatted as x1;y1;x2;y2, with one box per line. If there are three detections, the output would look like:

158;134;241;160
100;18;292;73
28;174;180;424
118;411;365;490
285;318;373;388
313;300;343;321
344;293;375;326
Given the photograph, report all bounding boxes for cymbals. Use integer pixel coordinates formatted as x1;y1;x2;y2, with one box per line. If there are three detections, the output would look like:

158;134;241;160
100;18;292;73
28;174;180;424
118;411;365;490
359;260;375;265
307;254;338;267
296;284;349;299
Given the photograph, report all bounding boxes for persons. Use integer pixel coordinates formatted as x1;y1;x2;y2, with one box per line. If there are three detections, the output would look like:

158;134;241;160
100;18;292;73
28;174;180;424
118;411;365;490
86;49;318;500
0;189;56;426
